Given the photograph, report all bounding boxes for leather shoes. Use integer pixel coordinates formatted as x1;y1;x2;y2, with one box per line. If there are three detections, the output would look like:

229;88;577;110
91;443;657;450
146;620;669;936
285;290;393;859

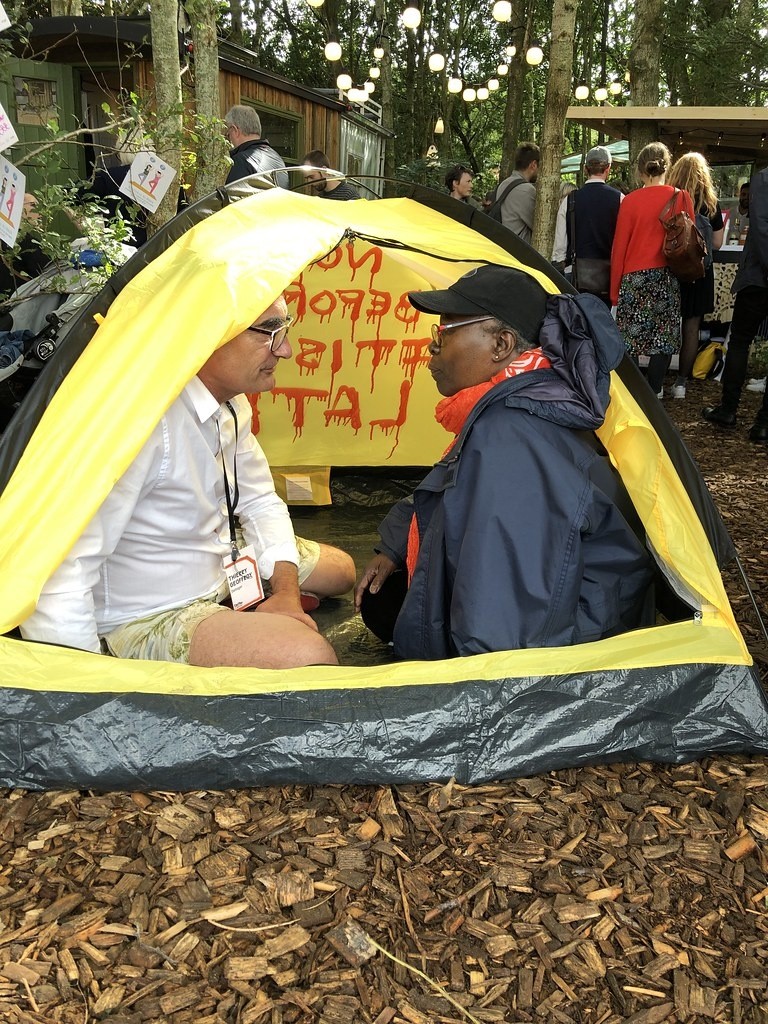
749;424;768;440
702;404;737;429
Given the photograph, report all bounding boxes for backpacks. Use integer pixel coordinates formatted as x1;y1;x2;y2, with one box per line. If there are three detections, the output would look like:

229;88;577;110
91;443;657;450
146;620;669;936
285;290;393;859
682;213;713;283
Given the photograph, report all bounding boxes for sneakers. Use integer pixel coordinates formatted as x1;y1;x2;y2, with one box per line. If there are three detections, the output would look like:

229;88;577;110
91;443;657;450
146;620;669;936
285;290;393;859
670;383;686;399
656;385;663;399
252;589;320;612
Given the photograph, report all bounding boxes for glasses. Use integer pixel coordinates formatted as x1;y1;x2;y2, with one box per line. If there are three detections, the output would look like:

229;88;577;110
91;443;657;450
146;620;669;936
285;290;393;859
430;317;495;346
224;126;233;136
246;316;294;352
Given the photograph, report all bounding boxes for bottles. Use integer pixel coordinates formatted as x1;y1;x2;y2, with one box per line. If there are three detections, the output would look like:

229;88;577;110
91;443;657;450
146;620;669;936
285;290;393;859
739;225;749;245
728;218;740;246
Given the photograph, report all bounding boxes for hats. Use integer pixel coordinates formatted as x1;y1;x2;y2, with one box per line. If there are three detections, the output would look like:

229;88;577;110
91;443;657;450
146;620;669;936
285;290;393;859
407;265;550;344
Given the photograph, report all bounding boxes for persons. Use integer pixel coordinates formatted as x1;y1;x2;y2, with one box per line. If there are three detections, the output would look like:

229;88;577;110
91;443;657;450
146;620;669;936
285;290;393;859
447;141;724;402
726;182;750;238
353;263;660;661
226;105;290;191
701;165;768;444
299;148;364;201
15;290;357;673
0;192;55;303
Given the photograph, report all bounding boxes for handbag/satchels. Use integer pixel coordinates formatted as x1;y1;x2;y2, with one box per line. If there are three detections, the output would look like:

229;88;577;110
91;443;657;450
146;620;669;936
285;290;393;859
563;257;611;294
658;187;708;280
692;339;728;380
485;179;529;224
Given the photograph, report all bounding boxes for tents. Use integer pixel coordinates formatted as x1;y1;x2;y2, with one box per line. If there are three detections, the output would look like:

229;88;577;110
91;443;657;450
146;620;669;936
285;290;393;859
0;163;768;791
